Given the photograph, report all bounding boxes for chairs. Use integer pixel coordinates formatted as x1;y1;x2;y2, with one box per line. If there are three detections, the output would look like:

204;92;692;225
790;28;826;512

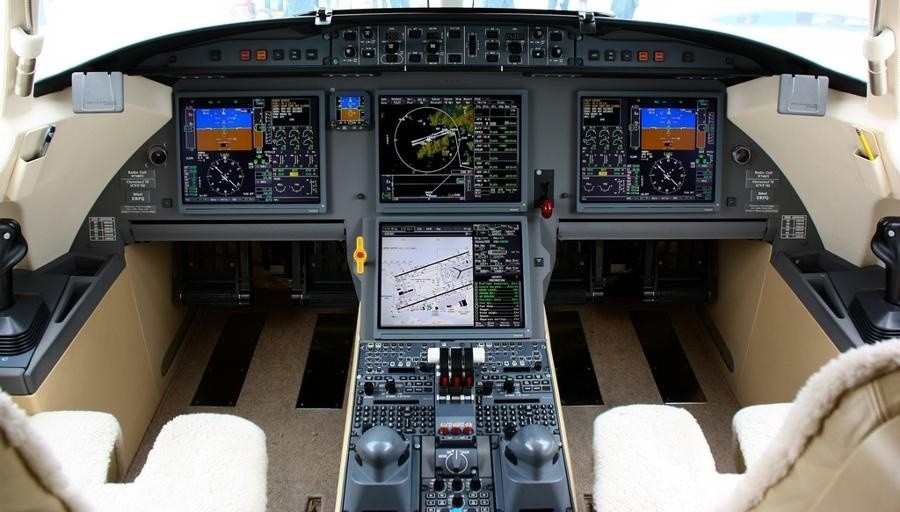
0;408;267;512
589;343;898;509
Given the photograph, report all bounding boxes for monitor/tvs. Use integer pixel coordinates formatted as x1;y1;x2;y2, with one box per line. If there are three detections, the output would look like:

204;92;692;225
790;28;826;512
575;88;724;214
173;89;327;216
374;88;529;214
373;216;533;340
